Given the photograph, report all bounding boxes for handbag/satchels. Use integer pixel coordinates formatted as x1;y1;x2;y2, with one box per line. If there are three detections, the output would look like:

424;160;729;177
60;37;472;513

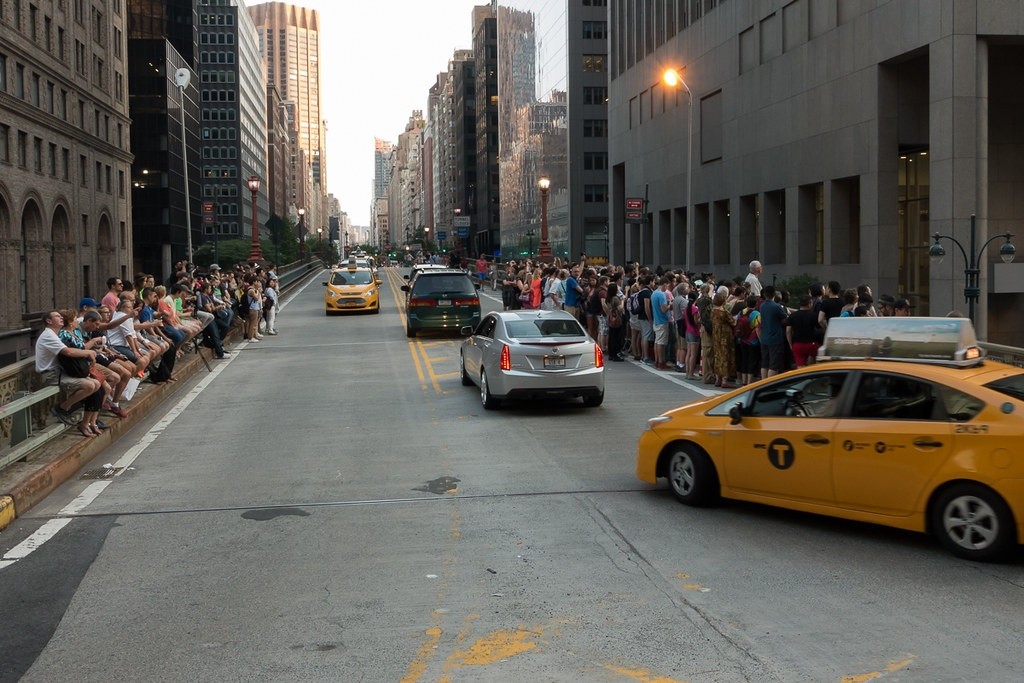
519;291;530;301
607;310;622;328
265;296;275;310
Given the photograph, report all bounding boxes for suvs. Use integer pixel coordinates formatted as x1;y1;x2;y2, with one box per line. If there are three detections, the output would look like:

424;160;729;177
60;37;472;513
401;262;481;337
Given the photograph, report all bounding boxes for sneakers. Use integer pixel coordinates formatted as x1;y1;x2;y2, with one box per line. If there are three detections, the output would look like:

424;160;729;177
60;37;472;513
655;363;687;372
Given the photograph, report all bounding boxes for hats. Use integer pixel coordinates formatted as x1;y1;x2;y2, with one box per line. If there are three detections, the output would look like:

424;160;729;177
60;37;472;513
877;294;896;307
894;298;916;308
210;264;222;270
79;297;101;308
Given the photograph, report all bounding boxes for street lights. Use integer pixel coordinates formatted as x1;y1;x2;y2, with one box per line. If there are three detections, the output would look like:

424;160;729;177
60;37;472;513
299;208;305;252
424;226;429;257
538;176;552;257
664;68;692;272
175;68;194;277
248;175;262;260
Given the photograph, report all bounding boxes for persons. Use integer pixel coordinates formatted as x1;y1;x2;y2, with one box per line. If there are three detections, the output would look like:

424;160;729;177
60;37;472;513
800;379;842;416
370;247;468;270
36;260;280;438
502;253;963;389
476;255;501;292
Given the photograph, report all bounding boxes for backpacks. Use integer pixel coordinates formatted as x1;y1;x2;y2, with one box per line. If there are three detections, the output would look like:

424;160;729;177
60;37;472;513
57;337;89;376
240;288;256;311
626;288;651;315
736;309;756;339
587;290;606;315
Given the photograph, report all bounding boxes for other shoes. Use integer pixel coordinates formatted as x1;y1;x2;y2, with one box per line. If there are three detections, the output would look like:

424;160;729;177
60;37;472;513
151;374;177;384
269;330;277;335
112;406;128;419
600;347;650;365
245;332;264;343
686;374;733;388
96;420;112;429
50;404;76;425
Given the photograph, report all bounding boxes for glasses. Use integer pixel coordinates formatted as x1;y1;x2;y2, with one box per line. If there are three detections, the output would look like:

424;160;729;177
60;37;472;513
53;315;61;317
103;311;110;313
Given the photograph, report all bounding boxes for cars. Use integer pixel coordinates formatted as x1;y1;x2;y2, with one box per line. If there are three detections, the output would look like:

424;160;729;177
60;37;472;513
636;316;1024;561
323;251;383;316
459;309;605;410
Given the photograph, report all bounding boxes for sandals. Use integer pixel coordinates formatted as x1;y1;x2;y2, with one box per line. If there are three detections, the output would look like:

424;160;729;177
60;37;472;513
78;424;97;437
88;422;102;435
